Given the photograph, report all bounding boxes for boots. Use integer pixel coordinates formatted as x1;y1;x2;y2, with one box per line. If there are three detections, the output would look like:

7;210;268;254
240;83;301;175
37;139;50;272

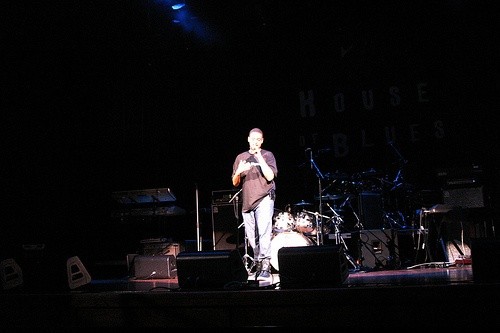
248;259;272;280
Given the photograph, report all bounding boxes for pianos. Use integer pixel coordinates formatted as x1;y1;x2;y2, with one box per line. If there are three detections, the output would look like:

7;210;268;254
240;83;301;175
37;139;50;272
111;206;186;217
112;187;176;204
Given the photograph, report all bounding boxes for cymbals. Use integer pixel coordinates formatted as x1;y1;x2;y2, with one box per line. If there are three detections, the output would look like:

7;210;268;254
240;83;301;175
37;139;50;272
295;202;314;205
324;208;345;212
315;195;340;200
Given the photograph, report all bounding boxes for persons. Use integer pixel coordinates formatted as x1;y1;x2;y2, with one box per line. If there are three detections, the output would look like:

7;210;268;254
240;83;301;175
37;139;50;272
371;280;500;333
231;128;278;280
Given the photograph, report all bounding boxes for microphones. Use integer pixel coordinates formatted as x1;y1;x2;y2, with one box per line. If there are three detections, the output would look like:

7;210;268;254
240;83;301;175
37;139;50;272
311;151;313;169
255;145;258;153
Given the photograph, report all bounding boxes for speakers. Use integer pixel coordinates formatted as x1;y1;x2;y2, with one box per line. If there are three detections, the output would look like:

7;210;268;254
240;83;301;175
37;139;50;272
175;249;248;290
59;250;92;290
356;229;400;269
278;244;349;289
212;203;245;251
471;238;500;284
0;258;23;290
134;255;177;279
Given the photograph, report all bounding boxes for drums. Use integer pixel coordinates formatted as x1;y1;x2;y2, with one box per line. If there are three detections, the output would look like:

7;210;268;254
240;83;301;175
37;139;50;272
307;218;331;235
295;212;313;233
273;211;294;233
269;231;317;271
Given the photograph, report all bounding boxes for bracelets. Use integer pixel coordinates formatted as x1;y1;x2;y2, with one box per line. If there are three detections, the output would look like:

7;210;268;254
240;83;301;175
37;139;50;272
233;173;240;176
